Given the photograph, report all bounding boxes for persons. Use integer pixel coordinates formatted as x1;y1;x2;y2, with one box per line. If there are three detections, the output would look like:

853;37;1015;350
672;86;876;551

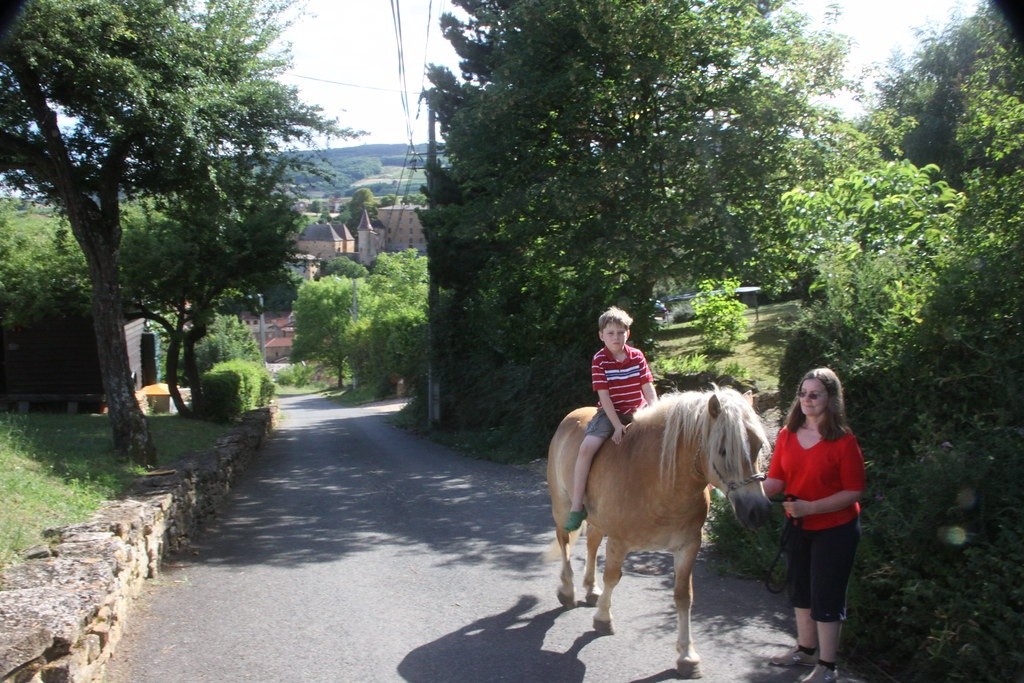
563;305;659;532
762;367;866;683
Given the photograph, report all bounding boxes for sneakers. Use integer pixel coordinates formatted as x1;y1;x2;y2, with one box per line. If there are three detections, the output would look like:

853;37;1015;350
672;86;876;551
564;503;587;532
800;663;838;683
770;647;818;666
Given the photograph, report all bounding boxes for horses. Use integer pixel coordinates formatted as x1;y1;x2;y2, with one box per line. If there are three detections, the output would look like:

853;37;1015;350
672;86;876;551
546;383;780;680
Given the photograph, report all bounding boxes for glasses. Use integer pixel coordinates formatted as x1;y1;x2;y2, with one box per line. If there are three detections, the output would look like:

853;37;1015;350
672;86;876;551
797;390;829;399
